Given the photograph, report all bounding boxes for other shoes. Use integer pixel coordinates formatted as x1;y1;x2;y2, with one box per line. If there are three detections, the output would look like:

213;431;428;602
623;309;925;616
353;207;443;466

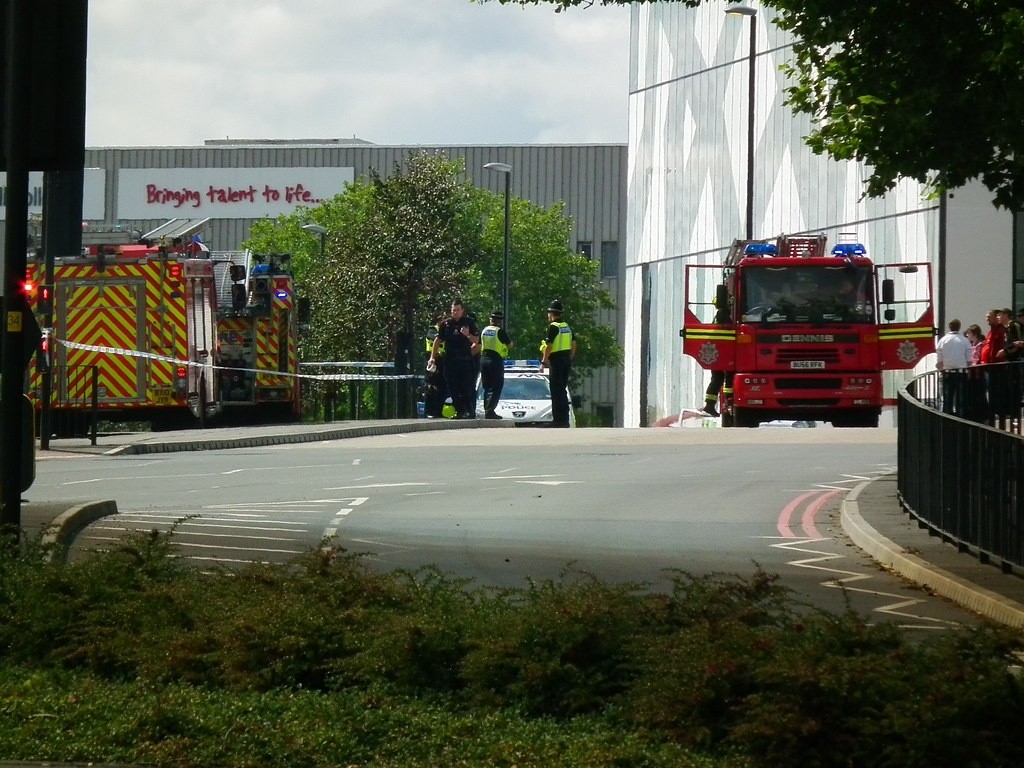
451;411;470;419
704;405;720;417
424;413;447;419
545;420;570;428
485;412;502;419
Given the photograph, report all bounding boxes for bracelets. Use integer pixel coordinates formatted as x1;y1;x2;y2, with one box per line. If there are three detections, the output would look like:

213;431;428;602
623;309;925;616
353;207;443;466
467;333;470;338
541;362;545;365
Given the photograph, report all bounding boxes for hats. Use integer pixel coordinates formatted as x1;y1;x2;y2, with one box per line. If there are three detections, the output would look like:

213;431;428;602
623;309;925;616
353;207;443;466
994;308;1013;317
1015;308;1024;316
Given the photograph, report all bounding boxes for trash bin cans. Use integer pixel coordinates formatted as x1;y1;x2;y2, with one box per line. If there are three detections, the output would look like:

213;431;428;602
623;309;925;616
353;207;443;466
596;405;614;428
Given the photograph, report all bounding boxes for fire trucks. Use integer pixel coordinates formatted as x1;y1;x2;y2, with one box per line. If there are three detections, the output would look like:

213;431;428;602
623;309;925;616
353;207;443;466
679;231;939;427
22;223;311;437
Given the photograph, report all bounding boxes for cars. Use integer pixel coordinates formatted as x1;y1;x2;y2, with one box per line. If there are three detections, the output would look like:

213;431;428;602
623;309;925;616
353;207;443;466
472;361;577;428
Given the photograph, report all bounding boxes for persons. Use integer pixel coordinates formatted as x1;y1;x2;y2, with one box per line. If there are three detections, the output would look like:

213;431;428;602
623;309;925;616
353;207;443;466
935;308;1024;427
539;300;577;428
703;295;734;417
834;280;866;307
480;311;513;419
424;299;483;419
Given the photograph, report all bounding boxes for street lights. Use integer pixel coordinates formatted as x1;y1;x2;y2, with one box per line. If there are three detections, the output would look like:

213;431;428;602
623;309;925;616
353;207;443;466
726;5;757;239
302;226;327;258
483;162;512;334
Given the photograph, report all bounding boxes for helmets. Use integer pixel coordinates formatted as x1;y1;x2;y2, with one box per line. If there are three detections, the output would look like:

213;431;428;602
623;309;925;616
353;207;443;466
467;313;477;321
546;299;564;314
713;294;731;307
488;311;504;319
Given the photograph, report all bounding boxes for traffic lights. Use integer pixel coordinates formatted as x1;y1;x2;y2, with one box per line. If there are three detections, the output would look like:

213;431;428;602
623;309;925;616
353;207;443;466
36;336;52;371
38;285;53;315
